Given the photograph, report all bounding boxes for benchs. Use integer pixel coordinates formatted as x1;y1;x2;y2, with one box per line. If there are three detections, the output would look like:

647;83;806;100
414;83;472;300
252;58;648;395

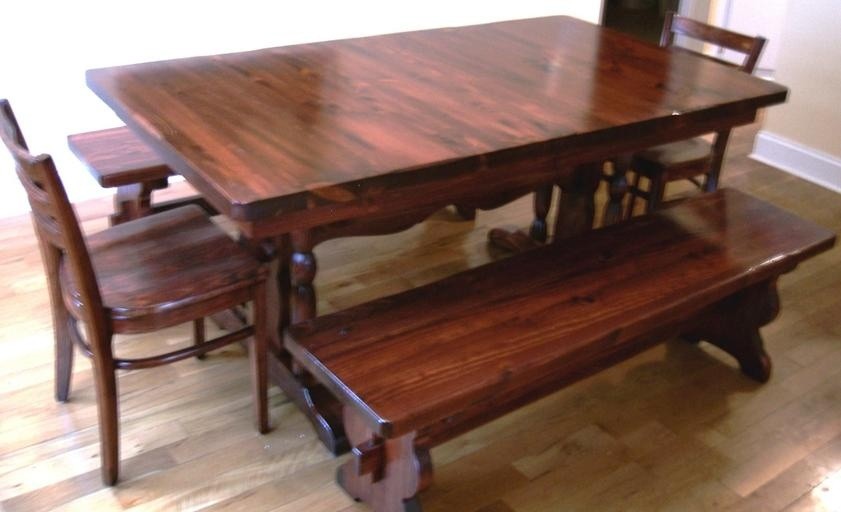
283;186;836;512
68;124;476;227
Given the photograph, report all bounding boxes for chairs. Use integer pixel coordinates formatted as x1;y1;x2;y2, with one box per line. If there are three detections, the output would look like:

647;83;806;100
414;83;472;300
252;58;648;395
0;99;270;486
601;12;766;221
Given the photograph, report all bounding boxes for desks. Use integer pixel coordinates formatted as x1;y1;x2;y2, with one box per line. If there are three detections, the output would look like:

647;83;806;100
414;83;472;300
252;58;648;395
86;15;788;455
